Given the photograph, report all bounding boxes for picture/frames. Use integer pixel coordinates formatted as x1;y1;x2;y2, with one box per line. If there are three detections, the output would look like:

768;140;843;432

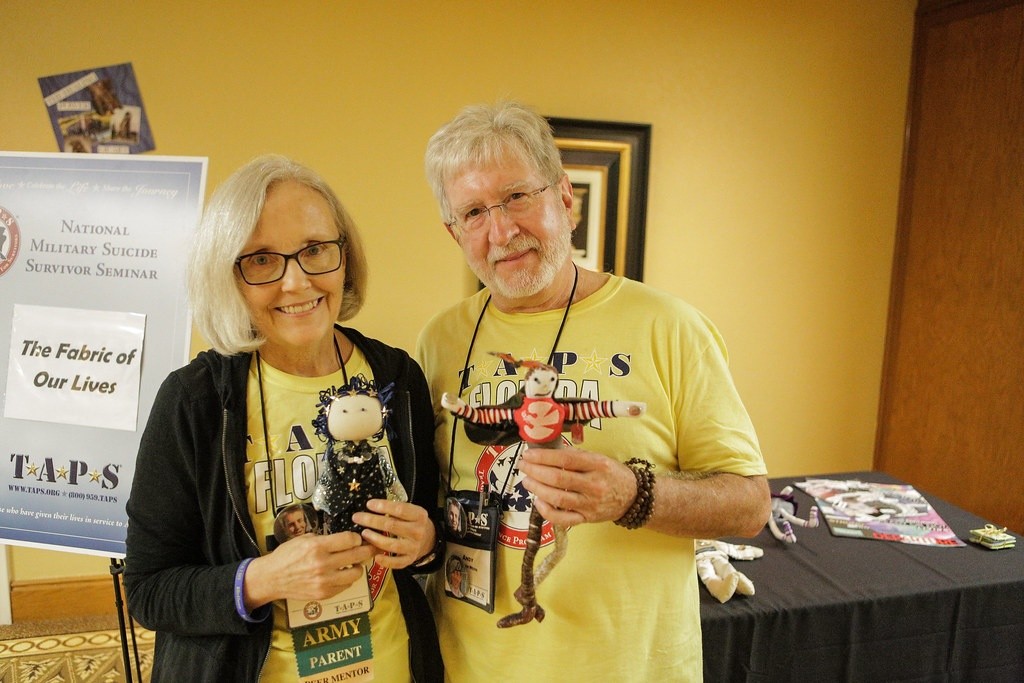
477;115;652;290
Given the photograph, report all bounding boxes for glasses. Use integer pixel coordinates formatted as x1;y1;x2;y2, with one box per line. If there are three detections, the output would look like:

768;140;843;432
449;184;549;233
235;236;342;285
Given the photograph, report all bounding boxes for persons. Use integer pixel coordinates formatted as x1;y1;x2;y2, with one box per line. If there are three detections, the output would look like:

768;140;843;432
449;561;463;597
282;506;306;538
417;102;772;683
447;499;460;531
121;156;448;683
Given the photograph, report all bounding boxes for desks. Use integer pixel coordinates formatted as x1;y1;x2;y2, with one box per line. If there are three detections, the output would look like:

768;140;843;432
699;471;1024;683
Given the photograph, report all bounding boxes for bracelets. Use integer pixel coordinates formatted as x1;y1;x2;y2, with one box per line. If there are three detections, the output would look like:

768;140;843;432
235;558;272;623
613;457;656;531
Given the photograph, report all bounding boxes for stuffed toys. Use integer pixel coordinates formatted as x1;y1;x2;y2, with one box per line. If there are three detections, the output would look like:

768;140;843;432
310;375;408;537
440;363;648;627
693;486;819;604
